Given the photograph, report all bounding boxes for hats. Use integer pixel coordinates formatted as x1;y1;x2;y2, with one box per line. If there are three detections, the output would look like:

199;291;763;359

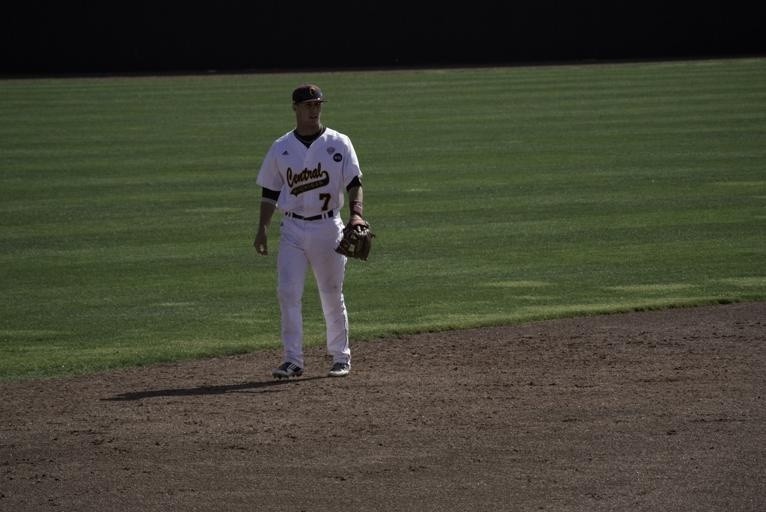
292;86;328;104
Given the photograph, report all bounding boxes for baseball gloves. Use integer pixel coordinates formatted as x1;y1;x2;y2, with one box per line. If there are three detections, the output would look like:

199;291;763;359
335;219;375;260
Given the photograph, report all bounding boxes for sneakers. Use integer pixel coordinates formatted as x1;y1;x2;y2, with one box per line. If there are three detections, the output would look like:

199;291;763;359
328;362;350;376
272;362;303;378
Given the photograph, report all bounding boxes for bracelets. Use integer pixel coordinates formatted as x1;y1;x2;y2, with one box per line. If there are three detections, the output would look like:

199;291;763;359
348;199;363;218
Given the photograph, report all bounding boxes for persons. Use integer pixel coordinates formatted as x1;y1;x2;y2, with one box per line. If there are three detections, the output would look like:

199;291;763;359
253;85;373;378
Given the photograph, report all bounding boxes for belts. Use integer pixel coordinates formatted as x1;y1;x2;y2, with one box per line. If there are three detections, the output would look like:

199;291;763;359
284;211;334;221
